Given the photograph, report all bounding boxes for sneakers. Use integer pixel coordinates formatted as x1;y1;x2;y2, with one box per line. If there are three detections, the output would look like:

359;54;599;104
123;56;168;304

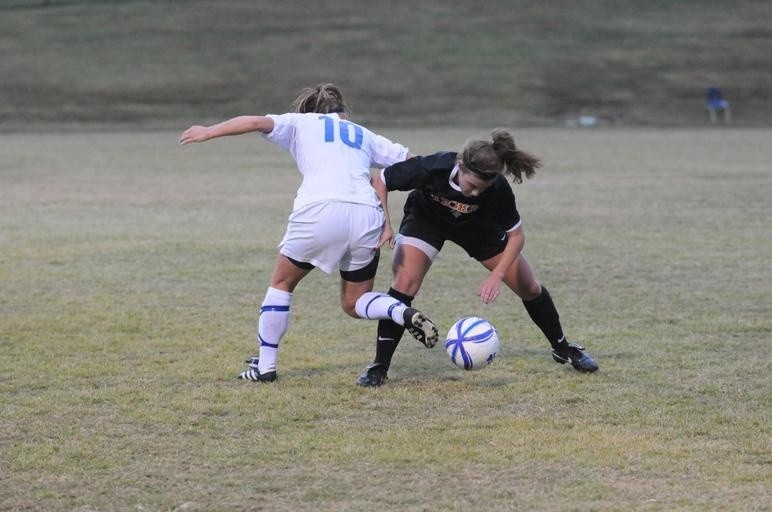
356;364;391;387
551;335;599;374
403;307;439;348
238;358;277;383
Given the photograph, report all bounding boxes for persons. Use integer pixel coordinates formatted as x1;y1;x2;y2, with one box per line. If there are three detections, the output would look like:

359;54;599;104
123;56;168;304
179;83;417;383
356;84;599;385
704;86;733;124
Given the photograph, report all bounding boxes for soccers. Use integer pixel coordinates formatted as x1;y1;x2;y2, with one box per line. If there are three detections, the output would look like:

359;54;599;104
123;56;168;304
445;317;499;370
